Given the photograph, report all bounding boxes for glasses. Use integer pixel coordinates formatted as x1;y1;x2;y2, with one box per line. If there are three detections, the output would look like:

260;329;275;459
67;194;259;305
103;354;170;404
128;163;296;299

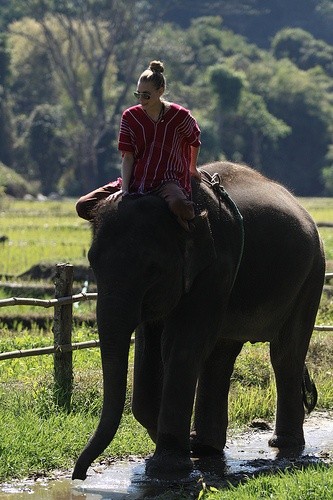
134;89;158;100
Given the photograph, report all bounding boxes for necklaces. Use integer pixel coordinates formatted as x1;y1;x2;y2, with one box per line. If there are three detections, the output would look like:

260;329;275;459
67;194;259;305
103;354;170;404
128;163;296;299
144;103;165;119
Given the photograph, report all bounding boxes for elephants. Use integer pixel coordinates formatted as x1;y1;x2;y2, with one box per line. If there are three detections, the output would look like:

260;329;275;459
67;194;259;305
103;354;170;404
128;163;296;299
68;161;325;481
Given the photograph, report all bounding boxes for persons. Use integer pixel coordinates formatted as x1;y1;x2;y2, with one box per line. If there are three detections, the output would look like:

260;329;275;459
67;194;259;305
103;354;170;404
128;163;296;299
76;61;220;262
